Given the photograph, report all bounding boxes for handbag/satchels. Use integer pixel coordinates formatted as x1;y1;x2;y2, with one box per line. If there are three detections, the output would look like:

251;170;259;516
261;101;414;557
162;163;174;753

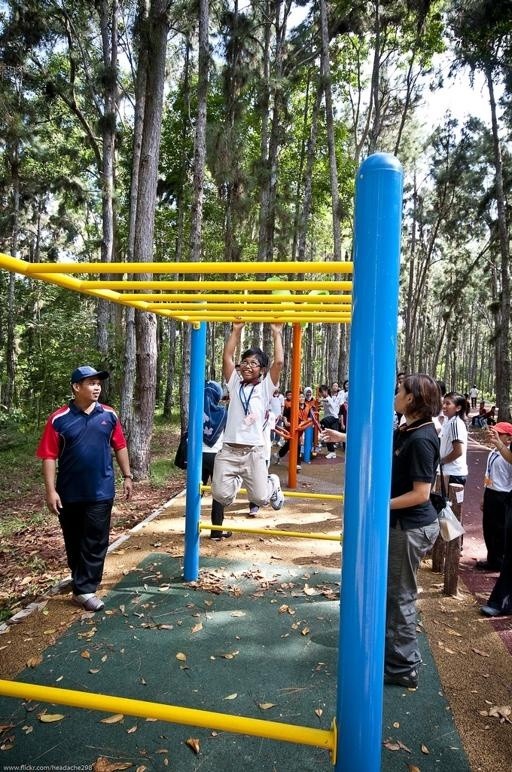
429;489;467;543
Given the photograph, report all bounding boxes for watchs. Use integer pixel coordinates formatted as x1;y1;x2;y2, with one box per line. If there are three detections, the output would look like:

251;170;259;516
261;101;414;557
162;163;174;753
123;474;135;481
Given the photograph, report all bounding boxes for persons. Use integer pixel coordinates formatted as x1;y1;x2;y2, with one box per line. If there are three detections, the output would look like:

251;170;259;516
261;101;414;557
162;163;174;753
36;365;133;613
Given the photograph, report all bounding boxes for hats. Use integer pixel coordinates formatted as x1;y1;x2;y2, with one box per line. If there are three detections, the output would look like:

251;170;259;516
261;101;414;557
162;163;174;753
489;421;512;437
71;366;109;383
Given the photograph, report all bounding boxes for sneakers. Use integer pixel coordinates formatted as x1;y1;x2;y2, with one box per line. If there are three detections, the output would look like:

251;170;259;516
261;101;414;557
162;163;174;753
476;559;501;573
71;590;105;612
479;603;505;618
249;502;261;516
267;473;285;511
296;464;301;470
325;451;338;461
209;528;233;540
273;450;281;465
384;670;421;689
